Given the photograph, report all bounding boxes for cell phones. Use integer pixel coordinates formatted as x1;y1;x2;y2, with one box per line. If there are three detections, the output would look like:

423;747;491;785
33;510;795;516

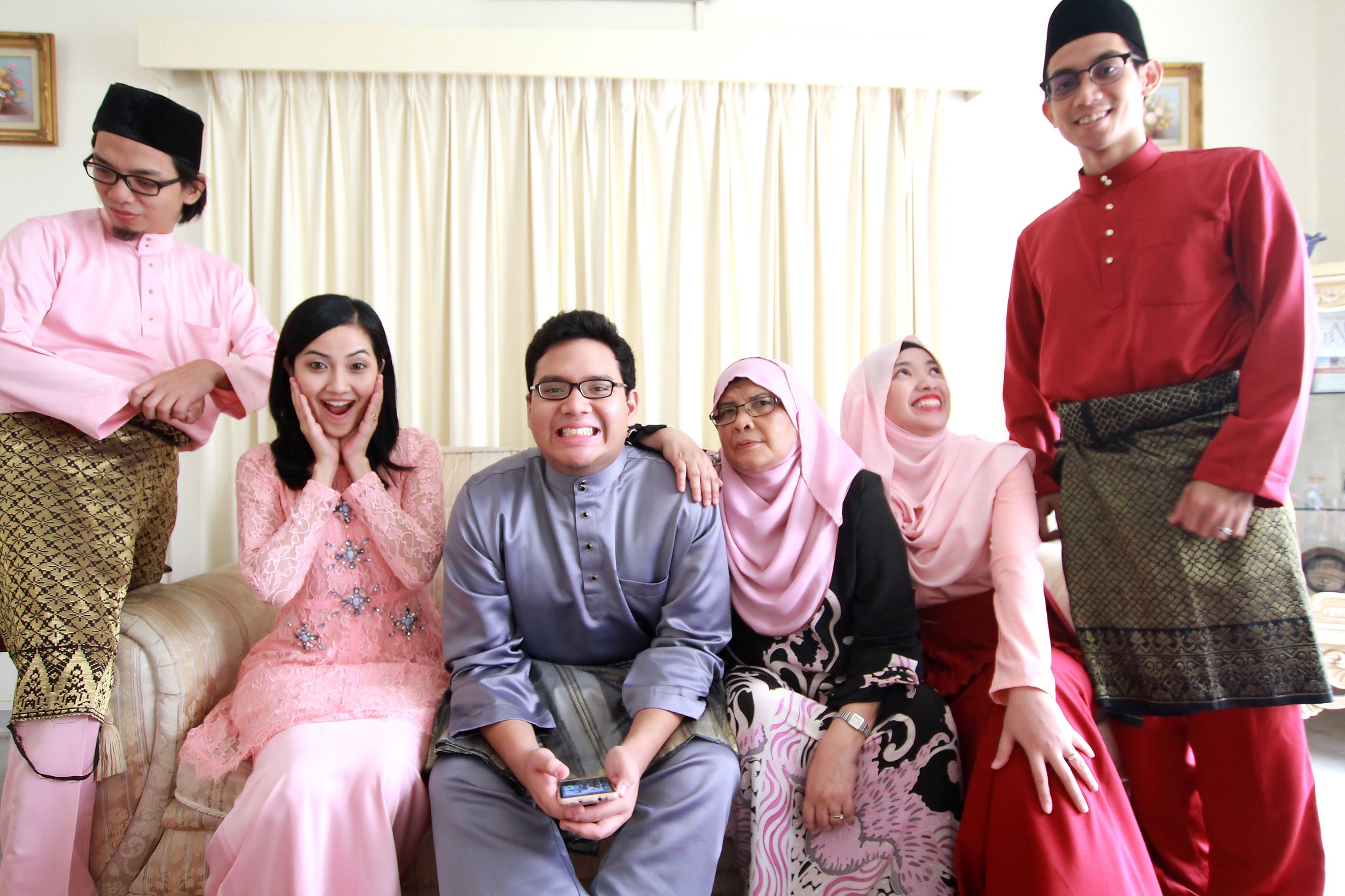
557;775;618;807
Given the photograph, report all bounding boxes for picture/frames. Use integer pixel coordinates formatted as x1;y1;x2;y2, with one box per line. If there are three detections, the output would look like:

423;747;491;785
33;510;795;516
1144;61;1204;152
0;30;57;148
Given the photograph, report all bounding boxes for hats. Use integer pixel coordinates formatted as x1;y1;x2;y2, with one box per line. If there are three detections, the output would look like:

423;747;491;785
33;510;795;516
91;82;204;171
1045;0;1151;64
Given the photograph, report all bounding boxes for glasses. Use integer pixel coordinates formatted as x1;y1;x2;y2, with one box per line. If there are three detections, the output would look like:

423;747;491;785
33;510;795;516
83;153;189;196
529;379;627;400
1039;51;1145;101
709;397;781;427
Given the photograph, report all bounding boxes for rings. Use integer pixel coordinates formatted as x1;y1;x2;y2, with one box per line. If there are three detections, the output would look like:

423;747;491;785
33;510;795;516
830;814;845;820
372;413;376;419
1067;753;1077;761
1215;524;1233;536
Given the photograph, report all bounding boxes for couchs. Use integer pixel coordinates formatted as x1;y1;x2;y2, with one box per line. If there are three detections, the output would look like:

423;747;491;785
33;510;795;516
86;450;736;896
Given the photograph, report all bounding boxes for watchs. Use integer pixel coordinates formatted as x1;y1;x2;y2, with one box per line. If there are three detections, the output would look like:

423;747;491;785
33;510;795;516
833;711;872;738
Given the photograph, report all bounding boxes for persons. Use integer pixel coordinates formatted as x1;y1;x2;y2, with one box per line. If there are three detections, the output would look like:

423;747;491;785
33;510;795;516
1002;0;1335;896
840;333;1165;896
180;294;452;896
625;357;961;896
430;307;743;896
0;84;279;896
